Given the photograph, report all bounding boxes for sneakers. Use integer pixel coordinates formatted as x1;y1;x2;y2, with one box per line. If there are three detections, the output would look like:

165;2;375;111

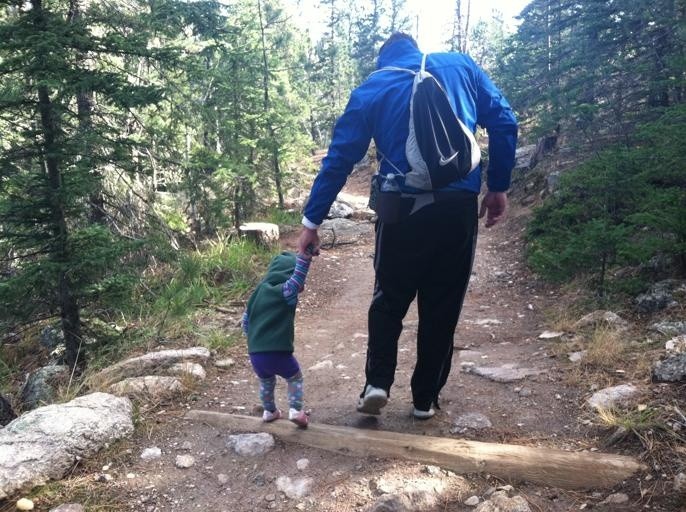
262;408;282;422
288;408;309;428
356;382;388;414
413;401;435;419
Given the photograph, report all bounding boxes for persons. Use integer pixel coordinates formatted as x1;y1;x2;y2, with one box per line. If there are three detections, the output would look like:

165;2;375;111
296;30;521;417
240;246;318;428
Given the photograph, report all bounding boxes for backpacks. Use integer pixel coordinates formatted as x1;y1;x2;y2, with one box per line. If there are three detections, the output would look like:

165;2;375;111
363;52;481;193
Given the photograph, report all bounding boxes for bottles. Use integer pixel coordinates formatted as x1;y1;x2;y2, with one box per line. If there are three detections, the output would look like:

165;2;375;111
380;173;402;192
